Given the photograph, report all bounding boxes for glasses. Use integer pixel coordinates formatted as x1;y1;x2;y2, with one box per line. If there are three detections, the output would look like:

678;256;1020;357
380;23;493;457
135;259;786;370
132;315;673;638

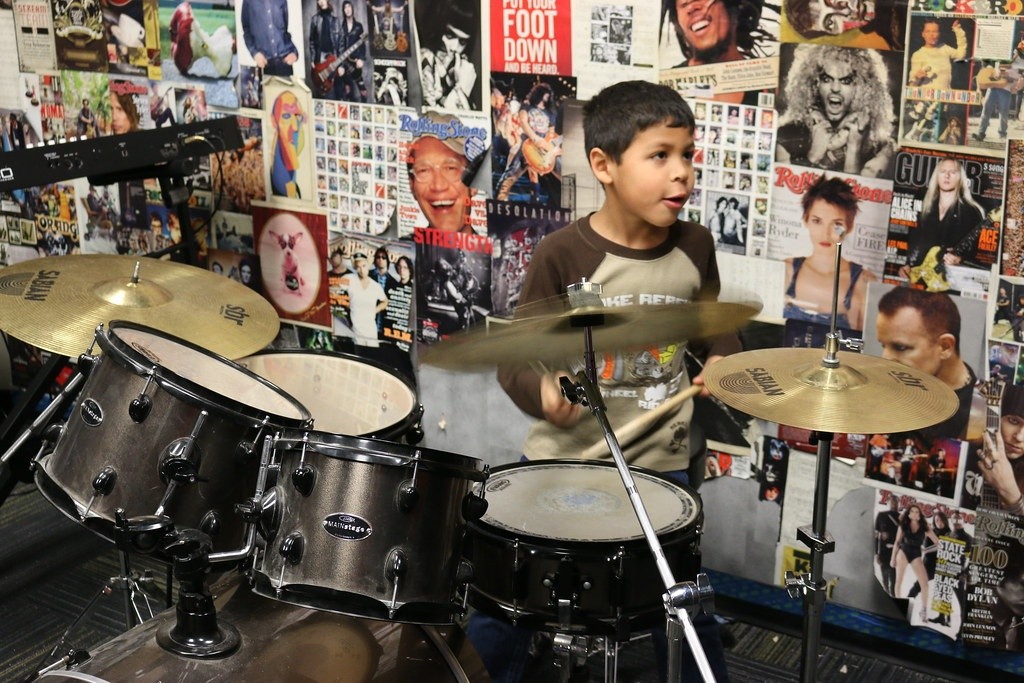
408;157;467;183
376;255;387;259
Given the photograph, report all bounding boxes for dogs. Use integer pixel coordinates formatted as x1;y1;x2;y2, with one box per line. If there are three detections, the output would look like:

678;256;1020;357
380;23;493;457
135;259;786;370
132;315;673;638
268;229;305;298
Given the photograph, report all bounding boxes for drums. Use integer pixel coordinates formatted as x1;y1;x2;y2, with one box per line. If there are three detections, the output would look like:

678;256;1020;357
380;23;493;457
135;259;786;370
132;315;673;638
29;572;491;683
231;349;426;447
251;428;485;626
33;319;314;570
465;458;705;630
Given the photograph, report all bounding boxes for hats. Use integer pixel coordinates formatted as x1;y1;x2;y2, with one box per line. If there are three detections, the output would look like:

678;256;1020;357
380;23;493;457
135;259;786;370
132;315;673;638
354;253;367;260
409;111;468;154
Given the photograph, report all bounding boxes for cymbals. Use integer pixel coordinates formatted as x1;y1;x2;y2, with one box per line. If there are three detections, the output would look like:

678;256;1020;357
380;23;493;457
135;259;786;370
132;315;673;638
702;347;958;433
0;254;279;359
418;297;761;375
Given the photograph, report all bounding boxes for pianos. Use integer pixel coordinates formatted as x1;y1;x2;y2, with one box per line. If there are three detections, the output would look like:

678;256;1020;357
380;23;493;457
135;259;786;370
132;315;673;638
0;112;245;196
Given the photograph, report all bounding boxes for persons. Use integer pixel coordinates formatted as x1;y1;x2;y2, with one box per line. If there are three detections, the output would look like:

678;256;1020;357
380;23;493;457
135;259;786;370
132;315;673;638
497;80;745;494
0;0;1024;655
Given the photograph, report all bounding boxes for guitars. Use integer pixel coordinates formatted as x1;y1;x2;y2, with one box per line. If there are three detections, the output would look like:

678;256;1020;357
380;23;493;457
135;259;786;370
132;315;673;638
312;31;369;91
872;446;903;457
900;453;929;463
372;15;420;56
909;205;1005;293
928;466;956;476
